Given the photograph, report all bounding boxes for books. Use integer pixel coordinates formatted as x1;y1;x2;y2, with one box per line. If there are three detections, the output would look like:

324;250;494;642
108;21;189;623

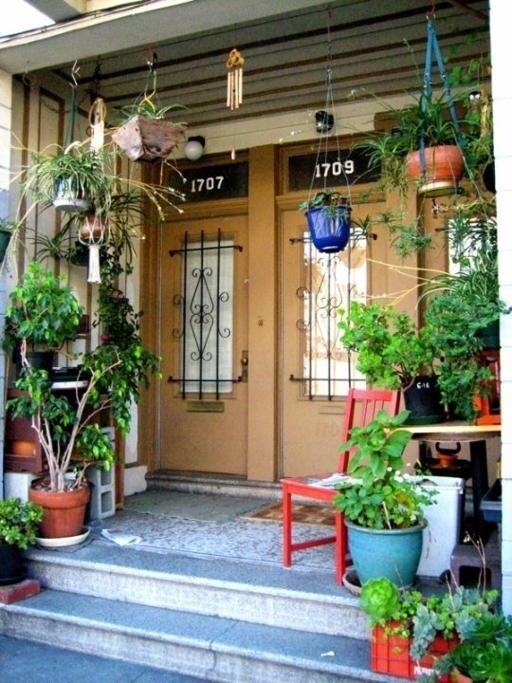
308;473;362;490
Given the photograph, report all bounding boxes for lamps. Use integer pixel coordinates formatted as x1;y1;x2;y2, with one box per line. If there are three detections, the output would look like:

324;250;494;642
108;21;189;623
186;134;205;160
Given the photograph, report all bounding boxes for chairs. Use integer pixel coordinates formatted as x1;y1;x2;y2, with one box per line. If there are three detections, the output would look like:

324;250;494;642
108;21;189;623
278;388;401;585
